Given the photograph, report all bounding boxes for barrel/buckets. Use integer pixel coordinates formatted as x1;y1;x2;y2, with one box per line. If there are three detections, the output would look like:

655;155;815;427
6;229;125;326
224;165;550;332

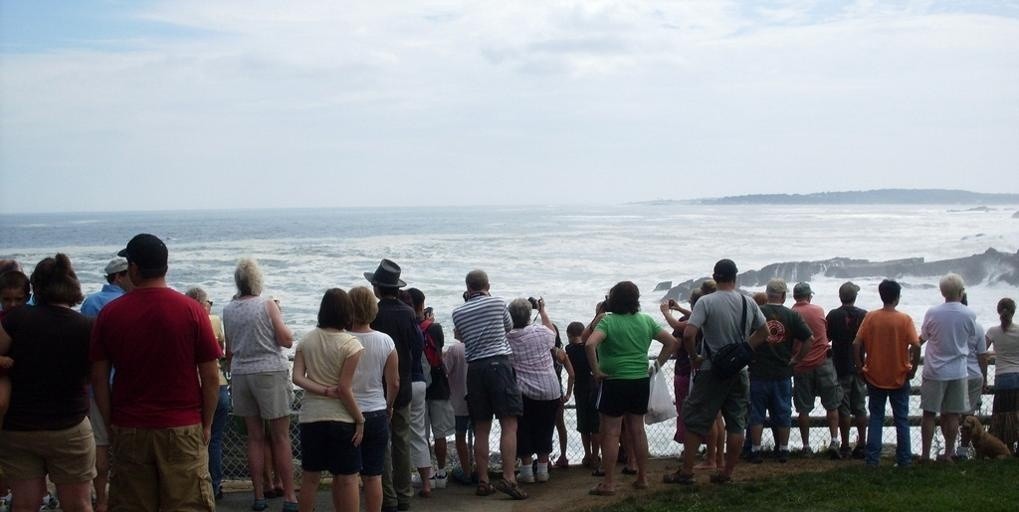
621;467;637;473
631;480;648;490
592;469;606;476
588;483;616;495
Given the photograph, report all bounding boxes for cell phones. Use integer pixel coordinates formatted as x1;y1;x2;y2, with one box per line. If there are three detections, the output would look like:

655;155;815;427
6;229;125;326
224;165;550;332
528;296;542;310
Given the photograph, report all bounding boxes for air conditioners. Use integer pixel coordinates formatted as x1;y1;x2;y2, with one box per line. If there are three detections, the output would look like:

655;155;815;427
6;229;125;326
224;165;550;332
962;415;1011;460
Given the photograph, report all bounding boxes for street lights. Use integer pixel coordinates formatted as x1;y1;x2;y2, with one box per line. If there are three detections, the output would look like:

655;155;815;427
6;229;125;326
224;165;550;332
713;344;748;376
426;364;449;401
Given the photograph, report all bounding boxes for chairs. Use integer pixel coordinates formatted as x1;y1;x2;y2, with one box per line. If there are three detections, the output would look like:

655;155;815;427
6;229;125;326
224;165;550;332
775;450;789;462
434;471;448;488
748;449;764;464
829;441;866;460
283;502;300;511
412;474;435;489
254;500;266;510
802;446;813;458
517;474;535;482
536;472;550;481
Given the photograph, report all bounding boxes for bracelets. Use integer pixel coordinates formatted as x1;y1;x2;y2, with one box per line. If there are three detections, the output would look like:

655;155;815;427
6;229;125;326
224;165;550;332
324;386;330;395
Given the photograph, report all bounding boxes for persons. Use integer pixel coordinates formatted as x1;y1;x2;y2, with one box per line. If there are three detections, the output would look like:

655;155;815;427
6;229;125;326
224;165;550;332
91;233;223;512
0;271;31;497
0;254;94;512
363;259;425;510
985;298;1019;455
660;259;771;486
581;282;680;497
223;259;299;510
1;259;37;310
80;257;136;512
790;282;840;460
342;286;400;510
919;273;976;464
452;269;528;499
395;288;456;499
291;289;364;510
550;323;575;466
825;283;868;459
740;292;768;457
186;287;229;499
442;326;472;485
505;296;561;483
853;280;921;469
744;280;815;465
565;322;601;466
910;293;990;460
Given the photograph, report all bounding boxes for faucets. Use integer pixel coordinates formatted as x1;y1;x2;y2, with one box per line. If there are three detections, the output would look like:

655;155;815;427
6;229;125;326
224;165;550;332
494;478;528;498
664;466;696;483
710;469;731;484
476;481;496;495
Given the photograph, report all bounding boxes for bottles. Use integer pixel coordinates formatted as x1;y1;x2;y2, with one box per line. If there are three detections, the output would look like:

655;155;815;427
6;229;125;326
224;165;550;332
105;257;128;273
118;234;168;269
766;278;788;293
840;282;860;298
793;282;815;296
363;258;406;288
715;259;737;276
